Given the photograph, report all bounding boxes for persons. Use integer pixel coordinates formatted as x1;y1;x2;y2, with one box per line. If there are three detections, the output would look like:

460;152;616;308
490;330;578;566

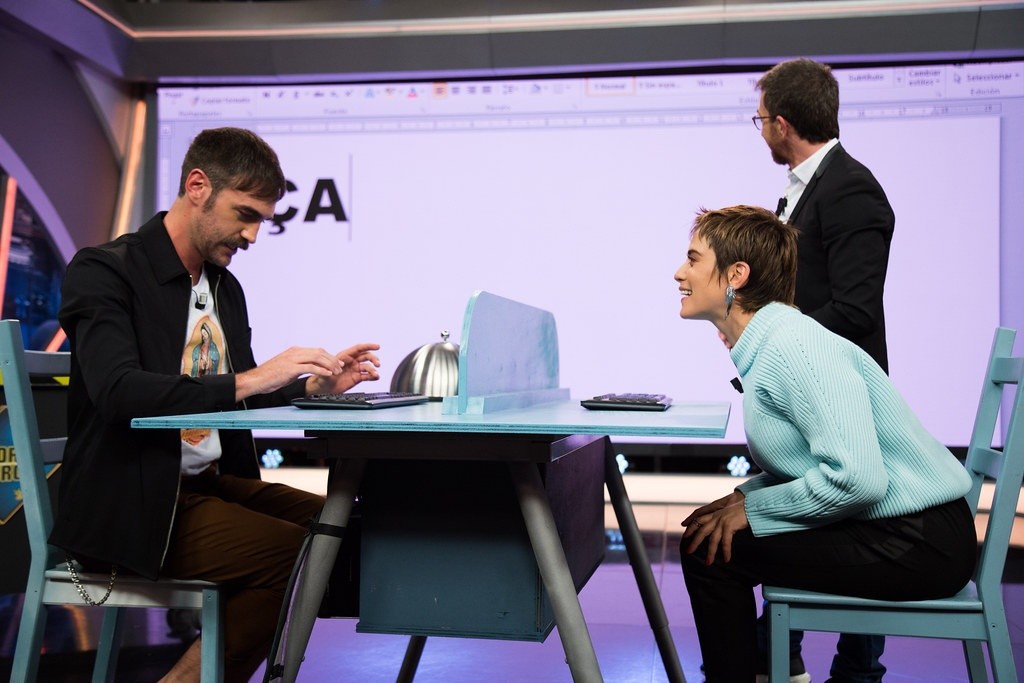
675;205;977;683
699;57;896;683
47;127;380;683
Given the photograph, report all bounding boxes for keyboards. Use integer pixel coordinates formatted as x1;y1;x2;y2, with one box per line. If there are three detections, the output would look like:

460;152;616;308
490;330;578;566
290;392;429;410
580;393;673;410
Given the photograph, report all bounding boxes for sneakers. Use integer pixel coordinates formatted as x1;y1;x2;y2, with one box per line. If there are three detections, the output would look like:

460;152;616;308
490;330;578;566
700;648;812;683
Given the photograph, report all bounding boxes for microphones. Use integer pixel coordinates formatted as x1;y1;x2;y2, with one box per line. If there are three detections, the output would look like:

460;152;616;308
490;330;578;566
730;377;744;394
195;301;206;310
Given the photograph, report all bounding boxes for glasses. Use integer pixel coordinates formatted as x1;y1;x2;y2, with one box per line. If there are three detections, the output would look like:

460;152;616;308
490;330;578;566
751;112;776;129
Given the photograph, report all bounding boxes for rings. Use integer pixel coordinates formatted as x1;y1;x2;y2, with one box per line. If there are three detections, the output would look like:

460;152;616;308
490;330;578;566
693;520;702;528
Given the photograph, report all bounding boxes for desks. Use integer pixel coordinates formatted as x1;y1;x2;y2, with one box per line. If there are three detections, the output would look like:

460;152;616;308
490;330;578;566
130;291;731;683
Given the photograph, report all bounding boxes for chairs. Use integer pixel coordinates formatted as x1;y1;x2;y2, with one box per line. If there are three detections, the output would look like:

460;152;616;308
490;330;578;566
765;327;1024;683
0;318;224;683
